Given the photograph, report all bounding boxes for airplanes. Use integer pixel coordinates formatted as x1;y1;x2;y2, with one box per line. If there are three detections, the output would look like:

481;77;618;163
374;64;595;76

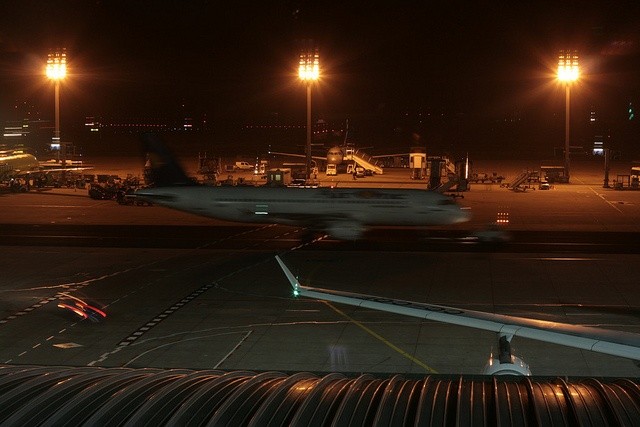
0;149;95;191
123;152;473;243
271;146;383;174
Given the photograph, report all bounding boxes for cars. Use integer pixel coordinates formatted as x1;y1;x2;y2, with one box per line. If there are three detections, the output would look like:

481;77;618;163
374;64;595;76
539;180;550;190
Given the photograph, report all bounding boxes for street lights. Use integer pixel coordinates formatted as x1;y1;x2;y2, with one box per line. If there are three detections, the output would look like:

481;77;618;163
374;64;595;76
298;47;320;177
45;47;68;163
554;48;580;177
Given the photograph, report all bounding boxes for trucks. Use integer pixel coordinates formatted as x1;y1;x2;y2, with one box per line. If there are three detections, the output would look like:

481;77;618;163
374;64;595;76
355;166;365;177
326;163;337;175
254;163;264;175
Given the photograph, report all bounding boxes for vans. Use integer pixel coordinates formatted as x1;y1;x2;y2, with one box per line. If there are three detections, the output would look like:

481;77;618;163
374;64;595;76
236;161;254;170
630;167;640;185
